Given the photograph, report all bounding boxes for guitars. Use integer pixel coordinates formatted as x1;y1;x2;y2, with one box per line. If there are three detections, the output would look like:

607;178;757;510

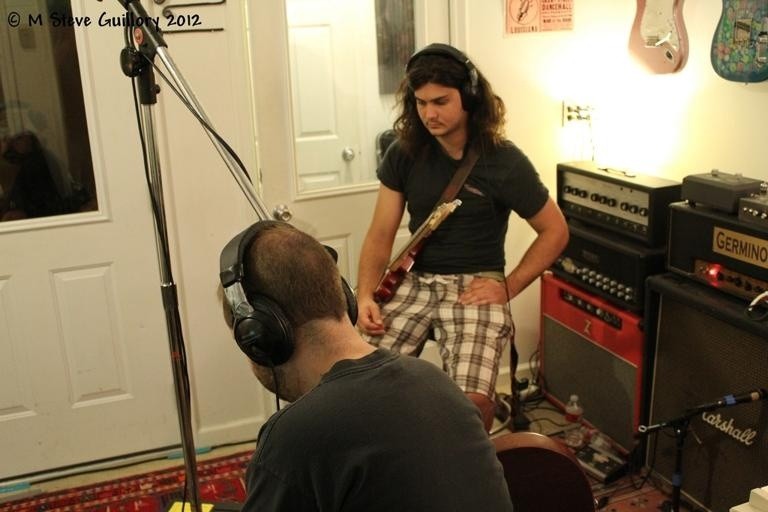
489;429;599;509
626;1;688;74
368;201;463;306
710;0;766;85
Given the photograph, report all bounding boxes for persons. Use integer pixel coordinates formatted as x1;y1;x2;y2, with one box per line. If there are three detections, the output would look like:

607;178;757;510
354;43;573;434
1;131;81;221
213;220;512;510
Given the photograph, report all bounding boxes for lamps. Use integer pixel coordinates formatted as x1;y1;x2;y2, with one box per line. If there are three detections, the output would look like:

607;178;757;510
552;90;596;164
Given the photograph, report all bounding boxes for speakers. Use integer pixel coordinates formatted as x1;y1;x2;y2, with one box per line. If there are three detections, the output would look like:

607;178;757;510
538;274;659;454
642;272;768;512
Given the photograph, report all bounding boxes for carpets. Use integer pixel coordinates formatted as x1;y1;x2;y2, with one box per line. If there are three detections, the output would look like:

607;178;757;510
0;365;767;511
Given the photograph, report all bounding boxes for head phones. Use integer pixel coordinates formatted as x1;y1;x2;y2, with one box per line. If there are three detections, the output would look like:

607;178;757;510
406;45;482;112
220;222;357;366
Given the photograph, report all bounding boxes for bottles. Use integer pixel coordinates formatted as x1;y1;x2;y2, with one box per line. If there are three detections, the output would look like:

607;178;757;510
563;394;584;447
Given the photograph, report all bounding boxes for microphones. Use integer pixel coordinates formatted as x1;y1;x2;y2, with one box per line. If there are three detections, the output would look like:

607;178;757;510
693;388;768;414
